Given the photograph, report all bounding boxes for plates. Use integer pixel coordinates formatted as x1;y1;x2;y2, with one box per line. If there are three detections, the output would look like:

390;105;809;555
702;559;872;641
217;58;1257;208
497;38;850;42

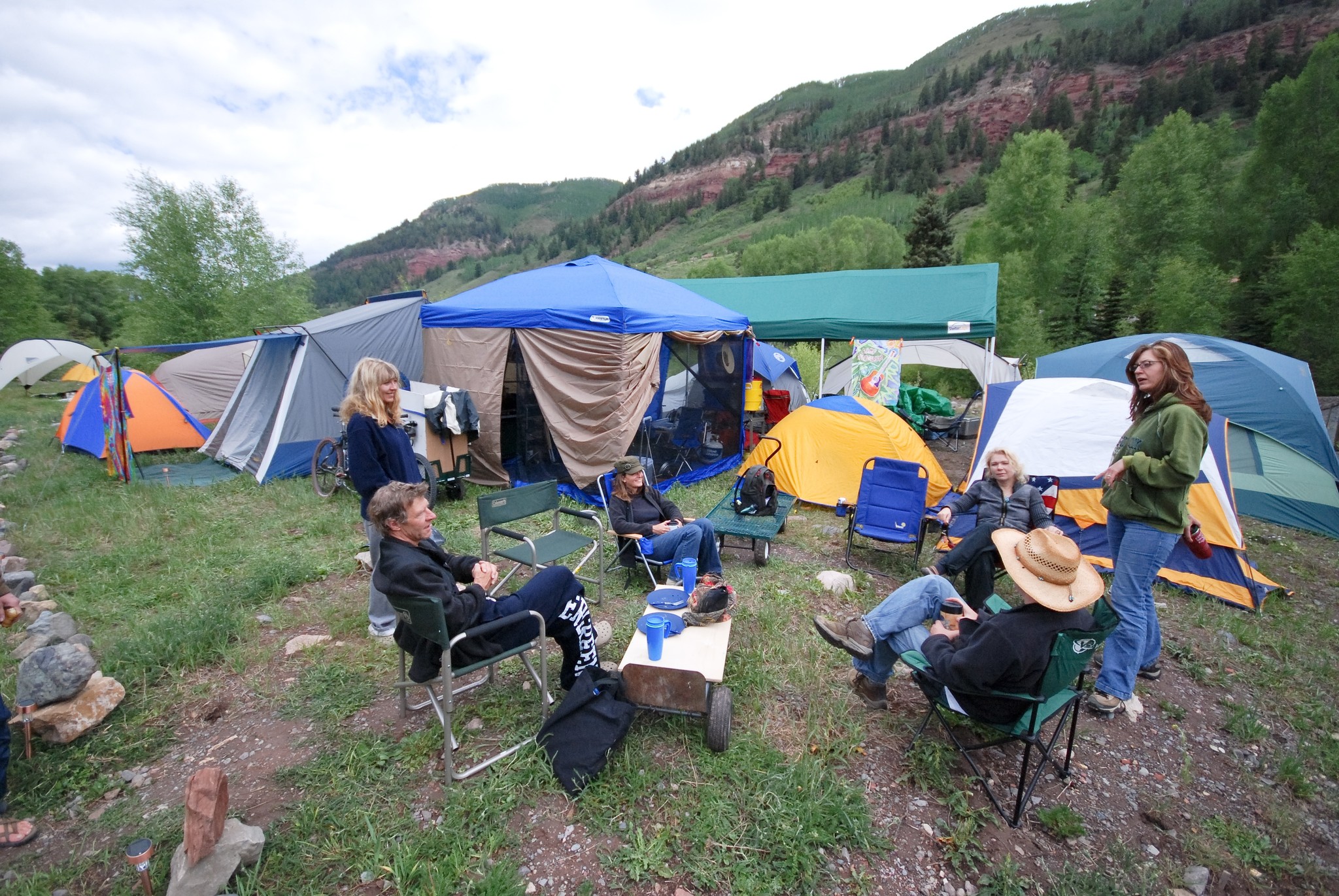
646;589;690;610
636;612;685;636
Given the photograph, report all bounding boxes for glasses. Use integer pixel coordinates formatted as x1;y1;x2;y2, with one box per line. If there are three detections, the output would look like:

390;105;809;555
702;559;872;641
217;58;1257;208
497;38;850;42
1129;360;1165;372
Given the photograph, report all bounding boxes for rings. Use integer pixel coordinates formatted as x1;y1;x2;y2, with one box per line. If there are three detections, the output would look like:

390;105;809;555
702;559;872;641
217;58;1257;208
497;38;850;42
1107;475;1111;477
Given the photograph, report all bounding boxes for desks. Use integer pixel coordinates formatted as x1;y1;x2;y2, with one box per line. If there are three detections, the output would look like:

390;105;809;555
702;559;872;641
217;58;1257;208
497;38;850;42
618;584;732;681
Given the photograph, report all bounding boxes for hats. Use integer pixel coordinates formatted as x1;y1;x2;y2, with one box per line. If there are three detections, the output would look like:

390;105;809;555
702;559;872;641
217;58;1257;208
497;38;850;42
991;527;1104;612
613;456;645;474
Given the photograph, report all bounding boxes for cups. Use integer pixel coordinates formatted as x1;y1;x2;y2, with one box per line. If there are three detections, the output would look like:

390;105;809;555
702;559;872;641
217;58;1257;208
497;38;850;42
666;520;678;531
835;497;846;517
674;557;697;593
646;615;671;661
1183;524;1213;560
939;600;964;631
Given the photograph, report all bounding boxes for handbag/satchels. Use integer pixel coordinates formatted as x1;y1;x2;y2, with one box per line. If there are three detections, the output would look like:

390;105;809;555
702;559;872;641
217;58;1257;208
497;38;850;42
538;665;638;797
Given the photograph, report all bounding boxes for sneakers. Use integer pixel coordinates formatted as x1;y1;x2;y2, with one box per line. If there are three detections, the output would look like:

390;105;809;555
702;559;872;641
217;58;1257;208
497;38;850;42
846;667;888;709
813;614;874;661
1085;687;1126;713
1093;653;1162;681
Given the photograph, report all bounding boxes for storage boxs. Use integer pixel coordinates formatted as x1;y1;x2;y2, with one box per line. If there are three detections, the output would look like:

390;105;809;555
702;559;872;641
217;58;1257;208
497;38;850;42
397;380;468;480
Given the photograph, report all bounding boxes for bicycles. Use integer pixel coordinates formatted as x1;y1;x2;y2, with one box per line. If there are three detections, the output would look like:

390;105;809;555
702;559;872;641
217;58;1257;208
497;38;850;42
311;407;436;511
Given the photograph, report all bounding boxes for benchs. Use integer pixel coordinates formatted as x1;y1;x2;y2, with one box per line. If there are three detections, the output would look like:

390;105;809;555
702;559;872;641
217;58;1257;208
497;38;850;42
836;457;929;579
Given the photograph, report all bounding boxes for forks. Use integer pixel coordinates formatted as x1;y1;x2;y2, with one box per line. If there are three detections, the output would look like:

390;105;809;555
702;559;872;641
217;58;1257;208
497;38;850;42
649;600;684;605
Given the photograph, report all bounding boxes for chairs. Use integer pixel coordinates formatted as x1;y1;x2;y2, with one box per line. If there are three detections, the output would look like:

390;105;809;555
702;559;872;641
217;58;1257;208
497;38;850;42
597;469;696;587
898;592;1121;829
897;408;962;452
385;595;548;779
477;479;604;607
661;407;706;477
943;468;1060;597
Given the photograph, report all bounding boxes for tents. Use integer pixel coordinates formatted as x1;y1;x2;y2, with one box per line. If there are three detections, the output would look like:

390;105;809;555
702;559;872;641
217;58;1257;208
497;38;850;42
92;288;432;486
1036;333;1339;540
734;396;956;512
656;261;999;400
418;255;760;509
953;377;1294;615
0;339;114;397
57;363;99;393
810;339;1028;403
44;366;212;458
147;339;256;424
662;340;810;433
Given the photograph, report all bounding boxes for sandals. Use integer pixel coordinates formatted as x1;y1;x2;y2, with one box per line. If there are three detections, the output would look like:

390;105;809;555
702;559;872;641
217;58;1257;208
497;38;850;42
561;661;618;697
921;565;939;575
593;620;612;649
0;818;36;846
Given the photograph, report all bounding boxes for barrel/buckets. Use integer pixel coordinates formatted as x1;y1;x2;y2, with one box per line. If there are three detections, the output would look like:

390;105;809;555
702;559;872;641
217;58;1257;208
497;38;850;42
744;376;763;411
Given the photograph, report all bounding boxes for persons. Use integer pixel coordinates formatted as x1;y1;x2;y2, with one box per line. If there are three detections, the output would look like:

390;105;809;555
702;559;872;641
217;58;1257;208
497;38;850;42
814;528;1122;727
337;357;423;636
609;456;723;586
1086;340;1211;713
366;480;618;700
921;448;1064;615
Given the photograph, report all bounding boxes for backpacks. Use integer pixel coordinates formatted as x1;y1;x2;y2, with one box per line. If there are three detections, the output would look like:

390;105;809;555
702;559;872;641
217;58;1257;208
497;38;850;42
730;465;779;516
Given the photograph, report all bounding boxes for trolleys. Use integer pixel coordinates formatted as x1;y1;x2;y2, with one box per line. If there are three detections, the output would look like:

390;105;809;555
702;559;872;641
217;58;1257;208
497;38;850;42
704;434;798;567
616;577;732;753
429;453;473;503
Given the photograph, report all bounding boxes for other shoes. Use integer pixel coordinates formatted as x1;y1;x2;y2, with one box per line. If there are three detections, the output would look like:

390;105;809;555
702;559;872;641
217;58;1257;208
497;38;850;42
368;624;398;646
